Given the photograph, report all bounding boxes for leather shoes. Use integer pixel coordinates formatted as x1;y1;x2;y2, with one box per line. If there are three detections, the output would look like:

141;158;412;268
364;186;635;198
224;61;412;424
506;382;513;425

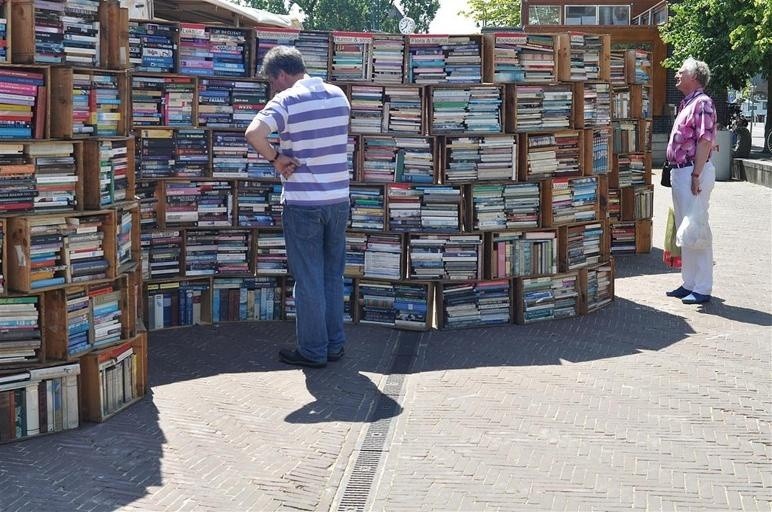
278;345;345;369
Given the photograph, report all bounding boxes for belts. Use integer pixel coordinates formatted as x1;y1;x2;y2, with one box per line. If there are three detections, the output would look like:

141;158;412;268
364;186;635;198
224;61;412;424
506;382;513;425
671;159;710;170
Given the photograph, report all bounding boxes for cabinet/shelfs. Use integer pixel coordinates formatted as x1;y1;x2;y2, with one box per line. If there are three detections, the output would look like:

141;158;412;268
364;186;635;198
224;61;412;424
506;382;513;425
1;1;149;447
130;19;653;333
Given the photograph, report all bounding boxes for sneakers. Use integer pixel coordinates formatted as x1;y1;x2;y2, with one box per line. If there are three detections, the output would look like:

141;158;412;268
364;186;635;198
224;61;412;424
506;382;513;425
665;286;710;303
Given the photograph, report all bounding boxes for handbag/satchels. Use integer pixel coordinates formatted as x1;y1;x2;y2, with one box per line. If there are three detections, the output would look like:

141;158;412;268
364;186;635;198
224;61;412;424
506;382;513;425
662;206;681;268
661;163;671;187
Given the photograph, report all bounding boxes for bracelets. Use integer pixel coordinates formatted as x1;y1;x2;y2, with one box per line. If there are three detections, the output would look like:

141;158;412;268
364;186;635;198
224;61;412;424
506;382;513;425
690;173;700;178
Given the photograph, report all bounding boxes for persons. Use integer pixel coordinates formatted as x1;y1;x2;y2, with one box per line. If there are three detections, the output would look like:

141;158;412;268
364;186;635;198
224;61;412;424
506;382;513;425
666;56;718;305
728;114;756;158
240;43;352;369
